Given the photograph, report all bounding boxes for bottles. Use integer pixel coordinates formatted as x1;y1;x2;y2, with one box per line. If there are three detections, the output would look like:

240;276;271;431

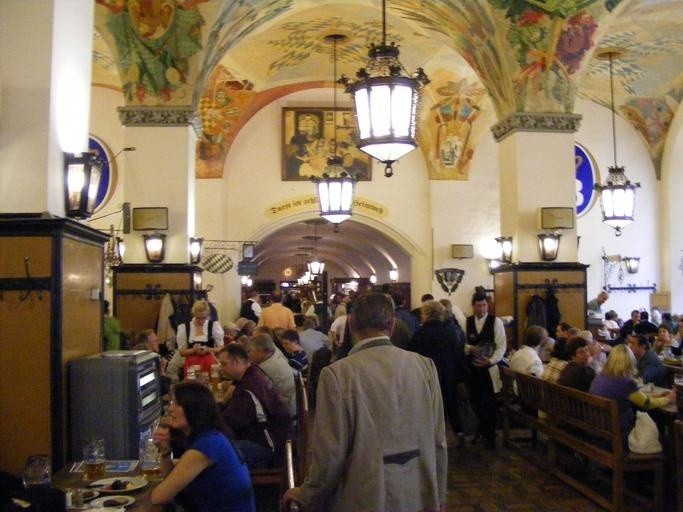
648;383;655;392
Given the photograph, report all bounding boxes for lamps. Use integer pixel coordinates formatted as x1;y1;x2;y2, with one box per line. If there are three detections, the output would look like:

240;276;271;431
625;257;646;276
142;234;170;261
316;40;356;231
191;239;398;289
537;235;561;261
593;57;641;234
63;153;109;218
497;238;516;262
343;0;427;176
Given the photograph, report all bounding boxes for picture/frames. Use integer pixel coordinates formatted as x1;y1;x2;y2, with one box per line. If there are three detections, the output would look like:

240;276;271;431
280;107;375;181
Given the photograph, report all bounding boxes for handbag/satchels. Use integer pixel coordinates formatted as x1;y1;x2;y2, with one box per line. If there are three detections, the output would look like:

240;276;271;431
628;409;663;454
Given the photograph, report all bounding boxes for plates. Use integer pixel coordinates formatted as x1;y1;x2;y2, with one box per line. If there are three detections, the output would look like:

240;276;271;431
70;476;148;512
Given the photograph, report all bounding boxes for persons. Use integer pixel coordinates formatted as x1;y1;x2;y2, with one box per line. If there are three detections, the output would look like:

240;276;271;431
138;282;508;512
509;291;683;496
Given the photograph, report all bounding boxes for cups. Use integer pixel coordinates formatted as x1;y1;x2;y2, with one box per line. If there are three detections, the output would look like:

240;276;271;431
22;455;52;489
82;438;105;479
188;365;225;401
140;439;161;472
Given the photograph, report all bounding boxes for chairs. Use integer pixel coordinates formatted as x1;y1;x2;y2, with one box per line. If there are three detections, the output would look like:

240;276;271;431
257;348;332;507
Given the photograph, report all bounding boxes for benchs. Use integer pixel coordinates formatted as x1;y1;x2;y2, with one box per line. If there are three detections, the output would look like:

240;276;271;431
498;365;663;507
